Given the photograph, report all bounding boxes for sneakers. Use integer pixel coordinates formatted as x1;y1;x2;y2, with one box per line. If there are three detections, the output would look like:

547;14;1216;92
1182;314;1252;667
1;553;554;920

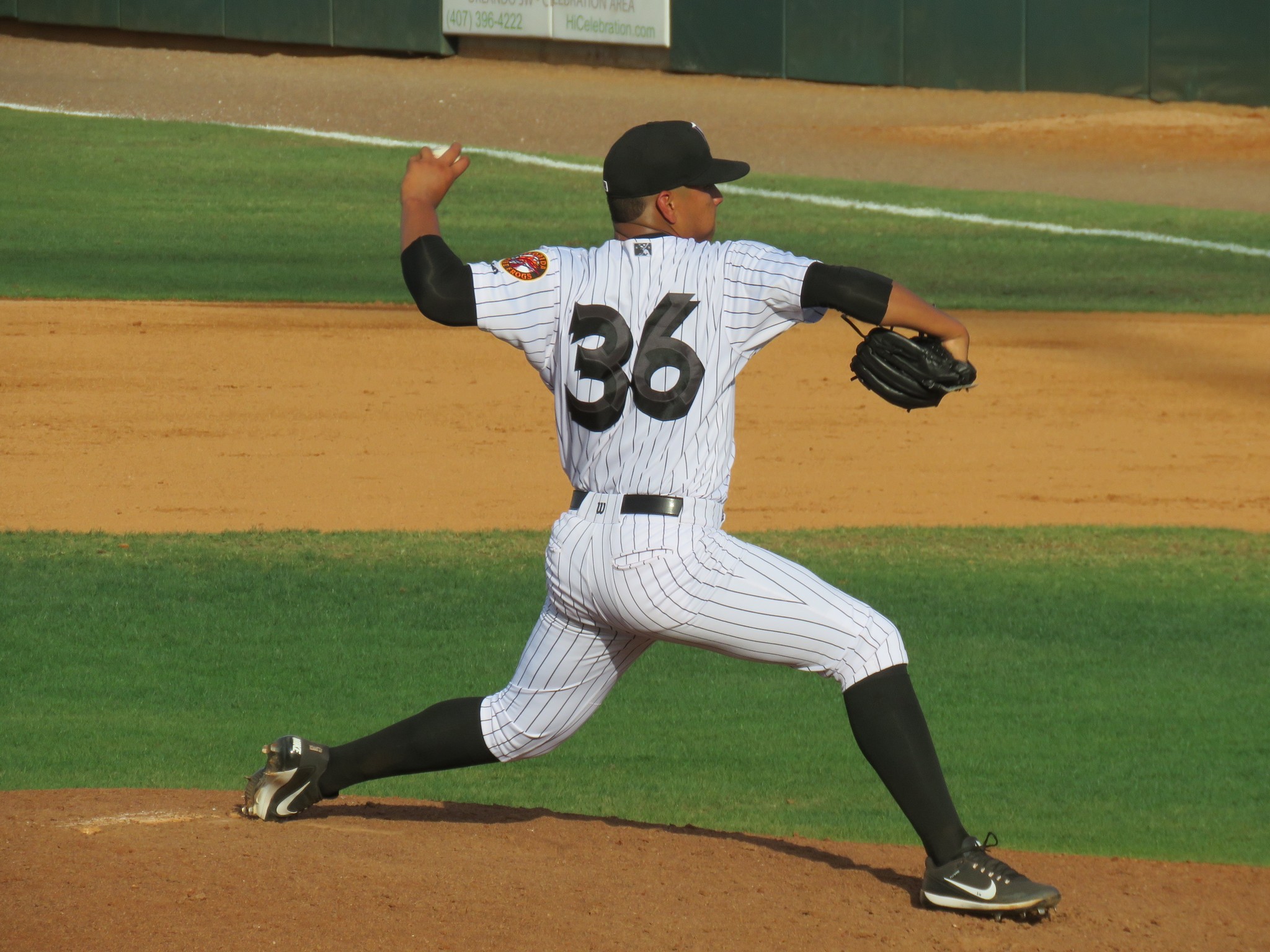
919;831;1059;919
241;735;338;819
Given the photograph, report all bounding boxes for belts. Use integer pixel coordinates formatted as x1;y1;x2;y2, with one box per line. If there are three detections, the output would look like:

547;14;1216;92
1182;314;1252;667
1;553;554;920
570;488;684;515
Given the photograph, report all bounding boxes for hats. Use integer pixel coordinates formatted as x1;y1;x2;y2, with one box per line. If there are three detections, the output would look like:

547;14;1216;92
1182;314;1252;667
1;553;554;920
601;121;750;195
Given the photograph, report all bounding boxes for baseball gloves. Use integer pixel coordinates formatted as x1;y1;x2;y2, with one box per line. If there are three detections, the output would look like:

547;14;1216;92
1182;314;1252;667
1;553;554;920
849;326;977;409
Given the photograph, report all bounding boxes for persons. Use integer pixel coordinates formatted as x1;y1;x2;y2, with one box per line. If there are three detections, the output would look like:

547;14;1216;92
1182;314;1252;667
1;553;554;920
240;120;1059;922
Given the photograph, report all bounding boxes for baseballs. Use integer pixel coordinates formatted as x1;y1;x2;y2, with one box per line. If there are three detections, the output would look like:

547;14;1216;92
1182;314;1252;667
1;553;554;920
432;145;461;165
